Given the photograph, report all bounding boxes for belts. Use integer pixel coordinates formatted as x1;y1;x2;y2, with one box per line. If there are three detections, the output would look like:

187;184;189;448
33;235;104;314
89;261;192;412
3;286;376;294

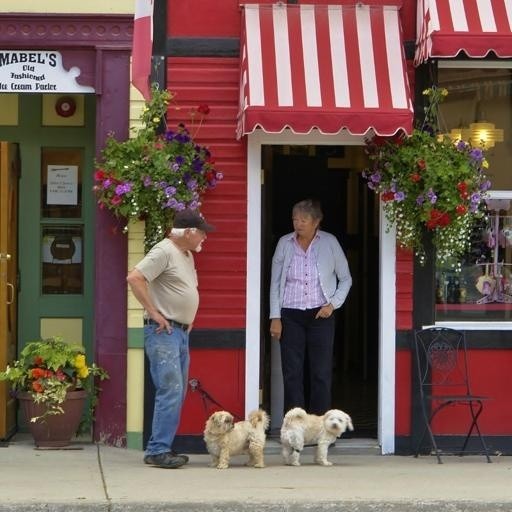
142;317;191;332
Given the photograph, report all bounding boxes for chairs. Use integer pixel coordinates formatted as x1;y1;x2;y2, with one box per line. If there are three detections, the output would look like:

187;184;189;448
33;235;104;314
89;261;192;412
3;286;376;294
412;324;495;465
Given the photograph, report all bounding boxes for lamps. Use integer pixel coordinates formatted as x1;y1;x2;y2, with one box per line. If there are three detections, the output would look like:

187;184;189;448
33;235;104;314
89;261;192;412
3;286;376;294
435;78;504;155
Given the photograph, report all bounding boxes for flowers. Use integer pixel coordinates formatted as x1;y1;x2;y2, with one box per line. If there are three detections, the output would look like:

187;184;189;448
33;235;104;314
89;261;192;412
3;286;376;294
89;74;225;257
356;83;495;294
1;333;114;441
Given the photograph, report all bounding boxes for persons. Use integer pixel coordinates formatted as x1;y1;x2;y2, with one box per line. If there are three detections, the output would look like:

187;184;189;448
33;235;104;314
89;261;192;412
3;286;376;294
269;200;353;448
126;207;215;468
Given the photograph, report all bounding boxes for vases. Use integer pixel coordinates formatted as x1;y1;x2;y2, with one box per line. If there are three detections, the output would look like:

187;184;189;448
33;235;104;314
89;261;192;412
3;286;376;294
16;387;90;450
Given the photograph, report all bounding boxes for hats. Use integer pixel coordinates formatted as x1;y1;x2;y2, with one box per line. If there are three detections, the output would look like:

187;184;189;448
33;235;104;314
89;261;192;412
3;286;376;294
171;207;218;233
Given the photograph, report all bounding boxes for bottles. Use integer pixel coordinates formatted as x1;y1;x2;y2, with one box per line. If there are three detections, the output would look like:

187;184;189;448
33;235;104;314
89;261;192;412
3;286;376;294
437;271;472;304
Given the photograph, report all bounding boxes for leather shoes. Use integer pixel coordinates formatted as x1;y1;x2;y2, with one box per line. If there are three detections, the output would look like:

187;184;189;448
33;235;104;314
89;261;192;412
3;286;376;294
142;452;187;468
171;451;191;464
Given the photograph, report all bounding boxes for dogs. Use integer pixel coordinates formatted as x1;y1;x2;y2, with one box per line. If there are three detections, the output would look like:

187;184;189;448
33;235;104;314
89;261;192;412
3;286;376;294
203;408;271;468
280;408;354;466
